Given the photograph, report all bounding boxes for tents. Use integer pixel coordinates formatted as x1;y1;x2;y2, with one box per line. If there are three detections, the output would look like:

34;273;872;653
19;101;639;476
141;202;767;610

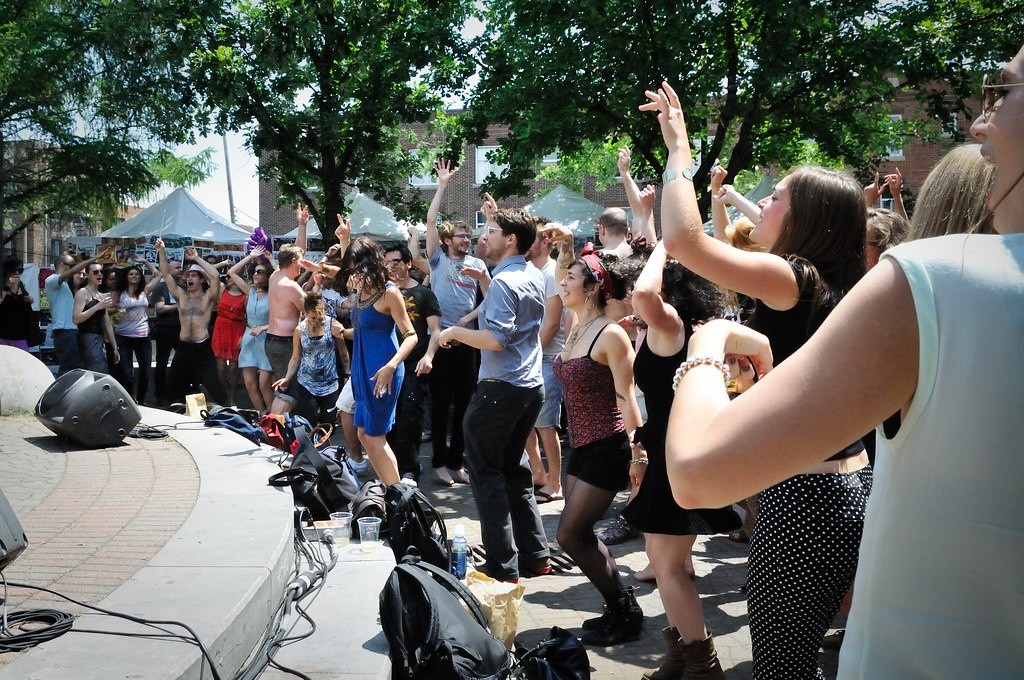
98;187;251;256
468;184;606;256
271;187;427;262
701;172;780;234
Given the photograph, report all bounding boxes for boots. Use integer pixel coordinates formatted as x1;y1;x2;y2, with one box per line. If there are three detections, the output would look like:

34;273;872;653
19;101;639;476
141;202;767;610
582;585;643;630
582;592;641;645
643;626;684;680
677;632;726;680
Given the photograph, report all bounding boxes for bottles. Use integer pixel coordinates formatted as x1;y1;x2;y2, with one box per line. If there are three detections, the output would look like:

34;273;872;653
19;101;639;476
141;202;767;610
451;526;467;581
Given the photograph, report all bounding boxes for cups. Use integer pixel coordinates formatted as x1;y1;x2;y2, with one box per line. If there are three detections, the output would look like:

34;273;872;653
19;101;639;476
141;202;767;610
118;302;126;316
103;292;111;301
329;511;353;545
357;516;382;553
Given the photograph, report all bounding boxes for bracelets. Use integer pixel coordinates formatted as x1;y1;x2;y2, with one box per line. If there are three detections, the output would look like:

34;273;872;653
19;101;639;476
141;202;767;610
673;355;731;394
630;459;648;464
324;252;330;259
662;168;693;182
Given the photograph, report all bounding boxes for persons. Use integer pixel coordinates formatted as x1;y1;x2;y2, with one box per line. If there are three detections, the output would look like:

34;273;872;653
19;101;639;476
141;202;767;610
1;136;997;642
638;81;872;680
437;207;551;584
665;41;1023;680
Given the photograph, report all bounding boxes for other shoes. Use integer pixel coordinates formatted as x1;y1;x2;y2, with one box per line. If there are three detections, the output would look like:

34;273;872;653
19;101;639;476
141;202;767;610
347;457;368;470
476;563;518;584
598;517;643;545
519;558;552;574
729;530;749;541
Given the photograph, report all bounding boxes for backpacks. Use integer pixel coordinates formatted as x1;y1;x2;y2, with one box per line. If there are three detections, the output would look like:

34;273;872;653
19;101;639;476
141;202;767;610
386;484;452;569
379;546;510;680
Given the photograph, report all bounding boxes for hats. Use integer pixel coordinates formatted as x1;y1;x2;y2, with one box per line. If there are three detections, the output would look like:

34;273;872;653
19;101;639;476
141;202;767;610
184;264;207;284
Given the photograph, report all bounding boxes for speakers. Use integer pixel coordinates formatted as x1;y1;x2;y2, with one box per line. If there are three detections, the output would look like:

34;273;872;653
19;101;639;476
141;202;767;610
33;368;142;449
0;488;28;573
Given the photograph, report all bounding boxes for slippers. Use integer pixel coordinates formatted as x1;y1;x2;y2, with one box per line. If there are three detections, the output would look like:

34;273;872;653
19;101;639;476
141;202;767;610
535;490;563;504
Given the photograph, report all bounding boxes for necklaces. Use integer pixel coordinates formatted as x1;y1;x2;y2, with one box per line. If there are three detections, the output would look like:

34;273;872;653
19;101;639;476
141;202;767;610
573;311;606;339
568;313;604;349
309;320;317;328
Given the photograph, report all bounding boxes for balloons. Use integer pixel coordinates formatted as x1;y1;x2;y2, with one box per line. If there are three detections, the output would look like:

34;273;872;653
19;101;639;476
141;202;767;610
247;227;268;253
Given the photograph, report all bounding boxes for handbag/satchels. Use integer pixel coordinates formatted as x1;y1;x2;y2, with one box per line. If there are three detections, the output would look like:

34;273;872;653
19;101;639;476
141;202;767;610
514;625;590;680
352;479;387;536
200;403;361;520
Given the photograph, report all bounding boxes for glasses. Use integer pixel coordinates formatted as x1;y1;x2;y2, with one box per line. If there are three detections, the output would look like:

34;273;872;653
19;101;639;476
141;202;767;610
10;267;23;273
450;234;472;240
392;259;403;264
94;270;103;275
979;74;1024;124
487;226;503;236
223;275;231;280
251;269;266;275
57;261;75;269
